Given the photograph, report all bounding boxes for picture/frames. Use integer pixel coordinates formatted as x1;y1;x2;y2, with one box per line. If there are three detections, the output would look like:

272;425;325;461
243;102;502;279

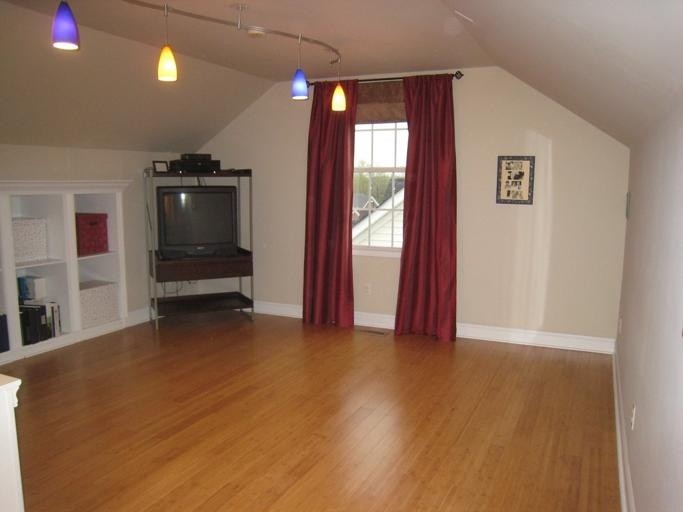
496;155;534;205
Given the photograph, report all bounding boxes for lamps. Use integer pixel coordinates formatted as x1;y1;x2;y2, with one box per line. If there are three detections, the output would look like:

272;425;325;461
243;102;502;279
290;34;347;111
156;3;178;83
52;0;79;52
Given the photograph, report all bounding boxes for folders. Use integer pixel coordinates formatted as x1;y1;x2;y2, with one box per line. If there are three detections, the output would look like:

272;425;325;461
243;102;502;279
24;305;48;341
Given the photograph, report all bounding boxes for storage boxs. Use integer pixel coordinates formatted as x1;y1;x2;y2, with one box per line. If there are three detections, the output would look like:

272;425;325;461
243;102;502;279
77;280;119;330
74;212;109;256
11;216;49;262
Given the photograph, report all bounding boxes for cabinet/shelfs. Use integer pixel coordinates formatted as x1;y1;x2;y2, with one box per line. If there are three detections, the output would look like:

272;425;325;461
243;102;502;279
0;179;136;369
146;169;254;331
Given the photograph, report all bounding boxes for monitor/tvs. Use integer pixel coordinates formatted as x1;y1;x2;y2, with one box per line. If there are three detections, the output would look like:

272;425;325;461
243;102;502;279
156;185;238;260
